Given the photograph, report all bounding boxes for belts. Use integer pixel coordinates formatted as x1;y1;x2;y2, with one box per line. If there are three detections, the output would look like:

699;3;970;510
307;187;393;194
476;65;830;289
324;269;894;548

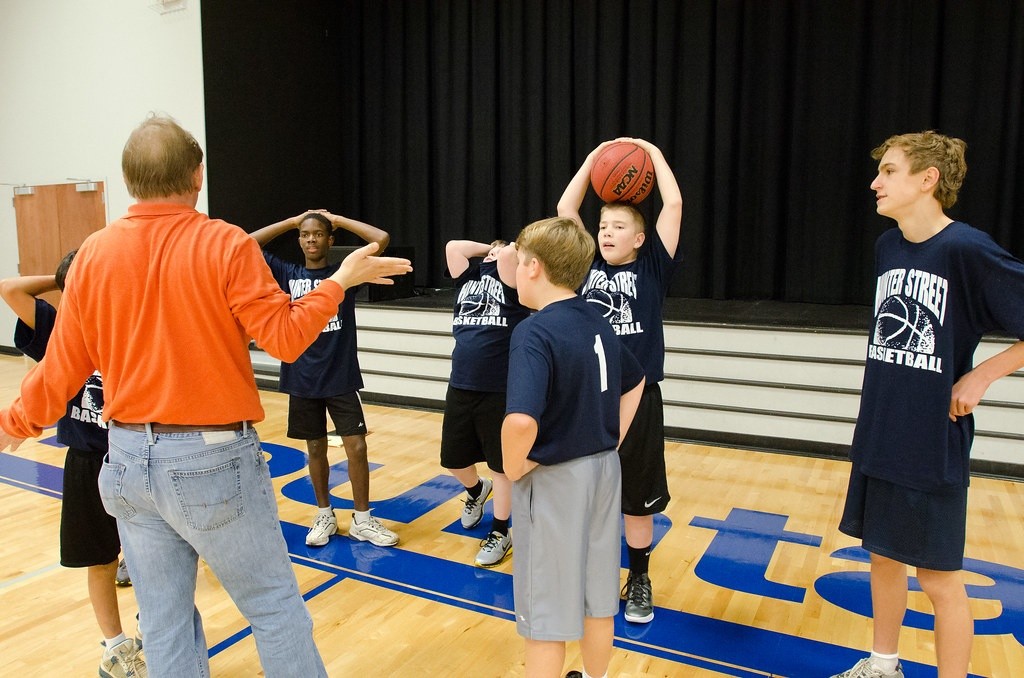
112;419;252;432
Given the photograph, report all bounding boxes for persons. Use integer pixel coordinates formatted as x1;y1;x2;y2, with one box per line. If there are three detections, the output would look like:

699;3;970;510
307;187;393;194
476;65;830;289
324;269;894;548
1;113;413;677
554;137;683;625
827;130;1024;678
499;218;648;678
2;249;149;678
438;238;528;569
246;208;403;547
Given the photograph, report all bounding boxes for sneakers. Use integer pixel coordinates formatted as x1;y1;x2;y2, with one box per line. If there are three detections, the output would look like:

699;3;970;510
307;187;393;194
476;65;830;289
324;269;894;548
829;658;905;678
460;476;493;529
116;565;131;582
475;528;513;568
305;510;337;546
621;569;655;624
348;513;399;546
99;638;148;678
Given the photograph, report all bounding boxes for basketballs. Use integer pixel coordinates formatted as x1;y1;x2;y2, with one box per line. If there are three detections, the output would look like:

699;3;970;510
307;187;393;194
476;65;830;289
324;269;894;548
590;141;655;204
